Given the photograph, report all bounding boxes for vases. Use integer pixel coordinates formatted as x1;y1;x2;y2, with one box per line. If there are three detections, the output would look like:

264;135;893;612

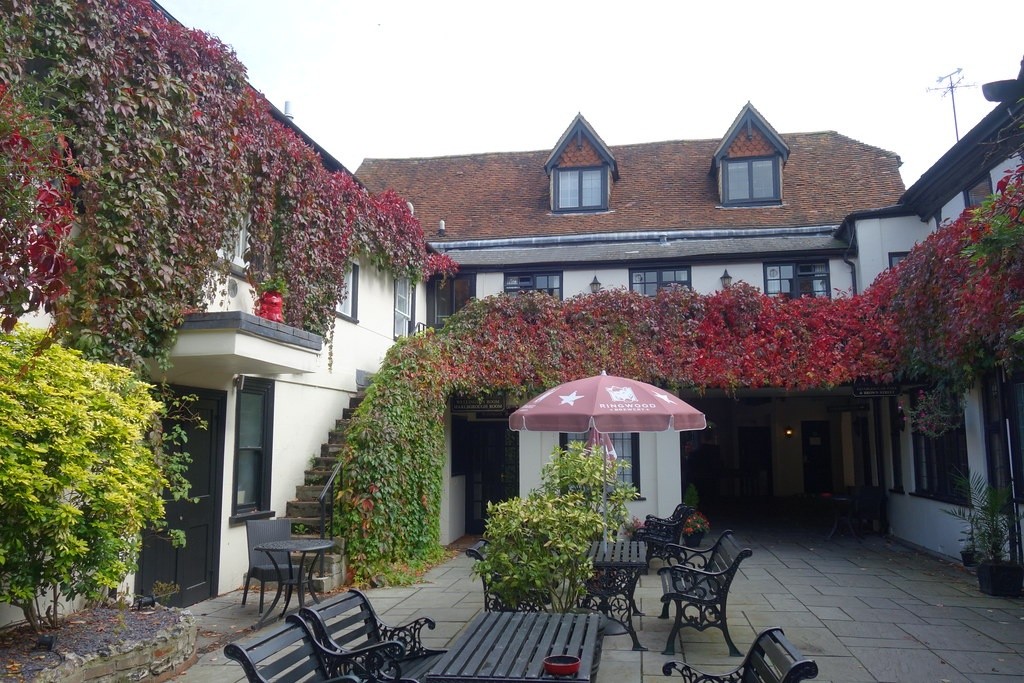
683;531;704;548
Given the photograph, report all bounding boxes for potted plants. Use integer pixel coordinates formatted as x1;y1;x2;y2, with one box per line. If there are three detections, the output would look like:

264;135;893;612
939;461;1023;599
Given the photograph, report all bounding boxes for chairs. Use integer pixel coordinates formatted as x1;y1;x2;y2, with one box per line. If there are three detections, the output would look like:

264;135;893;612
222;612;409;683
662;627;818;683
855;495;887;537
240;518;306;615
297;586;448;683
633;501;695;559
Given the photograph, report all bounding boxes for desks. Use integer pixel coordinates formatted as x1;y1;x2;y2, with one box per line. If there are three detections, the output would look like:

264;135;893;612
828;495;873;538
564;539;651;652
252;538;336;629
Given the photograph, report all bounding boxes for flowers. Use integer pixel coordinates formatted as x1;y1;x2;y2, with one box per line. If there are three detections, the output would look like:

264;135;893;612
682;510;710;536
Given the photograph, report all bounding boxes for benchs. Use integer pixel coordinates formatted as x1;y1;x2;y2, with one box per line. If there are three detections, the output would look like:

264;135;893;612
422;610;603;683
657;529;752;656
467;537;558;611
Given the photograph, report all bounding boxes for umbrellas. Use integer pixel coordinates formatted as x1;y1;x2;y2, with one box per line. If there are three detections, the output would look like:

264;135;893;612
586;427;617;501
509;370;707;553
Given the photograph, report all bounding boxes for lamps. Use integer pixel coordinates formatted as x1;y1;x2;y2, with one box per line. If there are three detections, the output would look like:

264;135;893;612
720;269;733;288
785;425;794;440
589;275;604;294
